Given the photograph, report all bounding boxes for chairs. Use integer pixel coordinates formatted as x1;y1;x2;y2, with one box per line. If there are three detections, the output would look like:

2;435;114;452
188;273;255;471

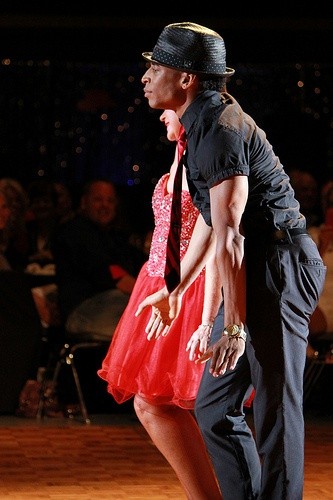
31;283;107;424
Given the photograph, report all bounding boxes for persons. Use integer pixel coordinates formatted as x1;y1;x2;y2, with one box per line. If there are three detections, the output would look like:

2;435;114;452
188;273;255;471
226;77;333;333
97;108;255;500
0;69;176;416
141;22;327;500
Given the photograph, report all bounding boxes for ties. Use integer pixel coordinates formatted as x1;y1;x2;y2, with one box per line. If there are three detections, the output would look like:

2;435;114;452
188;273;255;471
163;125;186;294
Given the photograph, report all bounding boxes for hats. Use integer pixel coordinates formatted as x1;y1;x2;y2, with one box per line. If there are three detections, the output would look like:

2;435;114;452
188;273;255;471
142;22;236;77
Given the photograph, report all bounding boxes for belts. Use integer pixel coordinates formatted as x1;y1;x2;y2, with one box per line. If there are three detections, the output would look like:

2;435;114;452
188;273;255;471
274;228;311;238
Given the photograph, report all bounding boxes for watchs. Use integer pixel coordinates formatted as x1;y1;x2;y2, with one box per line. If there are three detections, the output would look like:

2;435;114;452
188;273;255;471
221;324;247;341
